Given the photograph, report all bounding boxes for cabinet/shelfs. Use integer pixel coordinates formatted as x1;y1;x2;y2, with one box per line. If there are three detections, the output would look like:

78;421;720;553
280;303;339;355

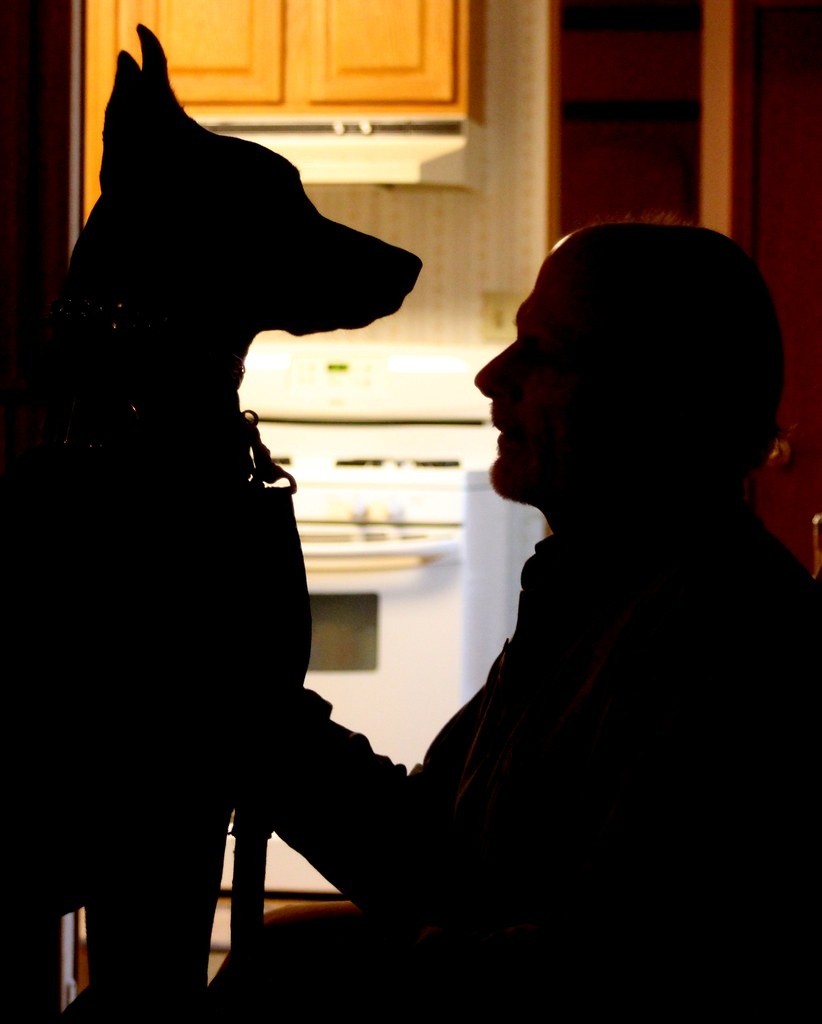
79;1;487;134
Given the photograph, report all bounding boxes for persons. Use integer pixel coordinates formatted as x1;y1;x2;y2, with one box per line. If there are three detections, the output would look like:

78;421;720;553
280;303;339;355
189;224;822;1024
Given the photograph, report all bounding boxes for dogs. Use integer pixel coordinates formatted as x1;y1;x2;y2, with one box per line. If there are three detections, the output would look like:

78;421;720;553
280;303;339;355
0;23;424;1024
